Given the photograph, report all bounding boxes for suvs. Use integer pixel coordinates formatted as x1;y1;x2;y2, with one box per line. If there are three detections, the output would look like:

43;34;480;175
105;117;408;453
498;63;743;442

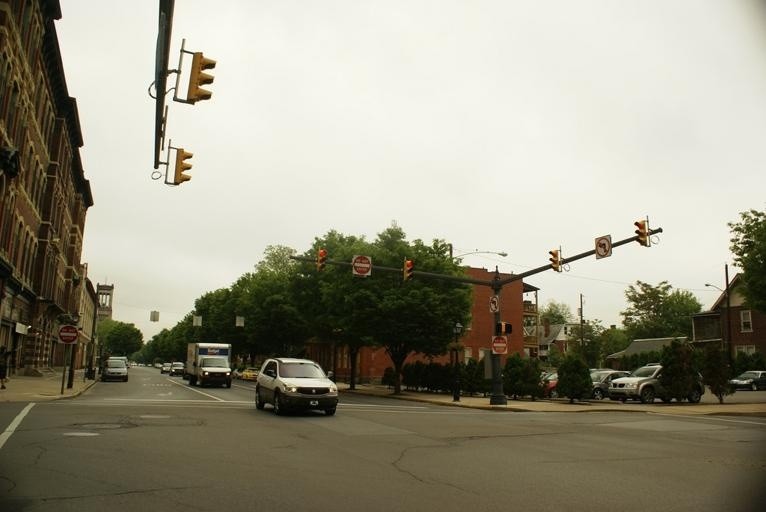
609;363;705;403
255;358;338;416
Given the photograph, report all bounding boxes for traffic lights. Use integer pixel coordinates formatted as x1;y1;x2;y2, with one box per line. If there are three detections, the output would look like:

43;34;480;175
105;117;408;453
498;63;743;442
174;147;193;185
318;246;328;272
548;250;558;271
186;51;216;102
635;220;647;246
403;256;414;282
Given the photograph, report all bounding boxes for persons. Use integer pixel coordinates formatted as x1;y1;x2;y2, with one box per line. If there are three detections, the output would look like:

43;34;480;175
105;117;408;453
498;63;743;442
0;344;18;389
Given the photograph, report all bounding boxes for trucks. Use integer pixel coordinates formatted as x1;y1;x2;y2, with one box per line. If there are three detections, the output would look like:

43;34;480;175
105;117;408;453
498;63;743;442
187;343;232;388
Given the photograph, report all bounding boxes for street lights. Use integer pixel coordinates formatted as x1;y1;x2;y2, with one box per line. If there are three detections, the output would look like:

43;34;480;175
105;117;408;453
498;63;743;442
449;252;507;258
452;323;463;401
67;310;81;388
705;264;732;377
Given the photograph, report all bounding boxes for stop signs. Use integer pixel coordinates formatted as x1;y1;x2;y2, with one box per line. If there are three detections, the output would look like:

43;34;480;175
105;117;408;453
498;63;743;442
352;255;372;275
491;335;508;355
58;324;79;345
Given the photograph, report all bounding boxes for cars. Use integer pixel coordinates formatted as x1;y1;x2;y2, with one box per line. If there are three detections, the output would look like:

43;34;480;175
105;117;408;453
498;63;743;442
102;357;130;381
729;371;766;391
539;369;632;400
161;362;184;376
233;368;259;381
